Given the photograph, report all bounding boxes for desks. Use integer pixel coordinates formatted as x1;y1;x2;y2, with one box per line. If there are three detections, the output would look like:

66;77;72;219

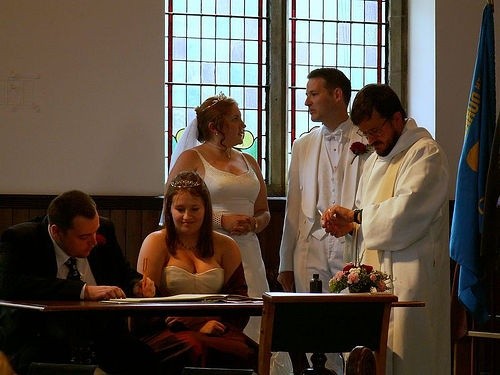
0;296;425;375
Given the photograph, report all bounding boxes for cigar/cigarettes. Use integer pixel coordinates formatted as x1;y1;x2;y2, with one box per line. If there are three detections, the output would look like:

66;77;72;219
318;210;322;216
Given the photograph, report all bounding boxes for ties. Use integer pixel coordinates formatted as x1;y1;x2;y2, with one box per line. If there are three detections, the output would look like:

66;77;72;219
64;257;81;281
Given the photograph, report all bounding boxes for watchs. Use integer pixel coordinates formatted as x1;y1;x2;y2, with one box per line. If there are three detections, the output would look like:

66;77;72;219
253;217;258;232
353;208;362;223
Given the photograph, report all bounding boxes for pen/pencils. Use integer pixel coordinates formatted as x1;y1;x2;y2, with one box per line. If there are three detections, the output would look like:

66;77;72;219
318;209;329;225
143;257;148;296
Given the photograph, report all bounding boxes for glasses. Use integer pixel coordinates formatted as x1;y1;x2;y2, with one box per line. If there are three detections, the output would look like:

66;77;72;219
356;116;391;137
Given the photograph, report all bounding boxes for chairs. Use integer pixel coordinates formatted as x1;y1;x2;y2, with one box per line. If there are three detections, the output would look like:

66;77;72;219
259;291;398;375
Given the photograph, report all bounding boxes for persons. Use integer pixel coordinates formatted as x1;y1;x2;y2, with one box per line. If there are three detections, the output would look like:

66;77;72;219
137;171;272;375
0;190;162;375
280;67;451;375
153;91;294;375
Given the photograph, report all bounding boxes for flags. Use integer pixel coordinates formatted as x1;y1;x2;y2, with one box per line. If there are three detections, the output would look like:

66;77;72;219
448;1;496;313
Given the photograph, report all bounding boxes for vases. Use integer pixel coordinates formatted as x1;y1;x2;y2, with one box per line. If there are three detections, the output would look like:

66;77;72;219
349;284;369;293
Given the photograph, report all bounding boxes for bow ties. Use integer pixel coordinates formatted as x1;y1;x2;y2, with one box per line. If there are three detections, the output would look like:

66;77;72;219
324;129;343;142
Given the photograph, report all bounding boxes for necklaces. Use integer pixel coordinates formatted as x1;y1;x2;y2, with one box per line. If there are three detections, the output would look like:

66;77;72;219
178;238;195;252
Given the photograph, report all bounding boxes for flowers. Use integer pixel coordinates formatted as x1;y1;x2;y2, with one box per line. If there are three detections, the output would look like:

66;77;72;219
349;142;371;165
329;261;395;292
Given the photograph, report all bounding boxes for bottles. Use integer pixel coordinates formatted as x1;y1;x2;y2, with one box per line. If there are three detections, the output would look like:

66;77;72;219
309;274;322;293
